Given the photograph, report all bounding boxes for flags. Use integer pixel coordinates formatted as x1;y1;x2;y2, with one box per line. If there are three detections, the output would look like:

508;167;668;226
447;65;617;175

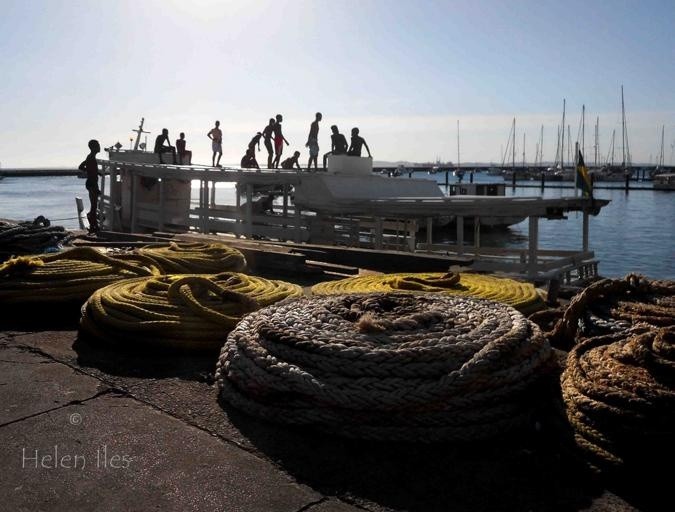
575;149;592;199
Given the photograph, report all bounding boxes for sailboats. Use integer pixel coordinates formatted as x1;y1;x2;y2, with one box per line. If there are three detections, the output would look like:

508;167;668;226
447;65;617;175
455;84;671;181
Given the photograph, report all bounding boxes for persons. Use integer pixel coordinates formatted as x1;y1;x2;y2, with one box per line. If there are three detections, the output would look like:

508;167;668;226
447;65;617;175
323;125;348;171
281;150;301;168
304;112;323;171
154;128;179;164
240;114;290;170
347;127;373;159
207;121;224;167
79;139;101;230
176;133;193;165
240;192;278;241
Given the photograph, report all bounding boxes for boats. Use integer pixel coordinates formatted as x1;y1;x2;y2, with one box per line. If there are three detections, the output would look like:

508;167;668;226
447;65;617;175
77;117;602;281
